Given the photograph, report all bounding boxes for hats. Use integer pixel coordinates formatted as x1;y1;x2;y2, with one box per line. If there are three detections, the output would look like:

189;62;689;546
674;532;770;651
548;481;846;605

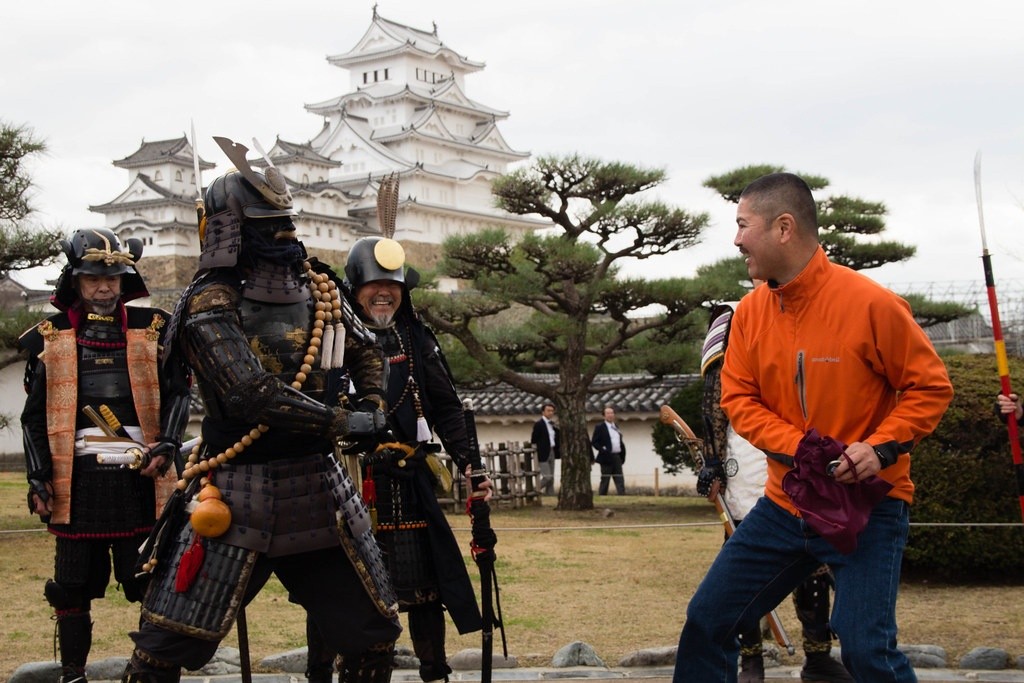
699;310;732;381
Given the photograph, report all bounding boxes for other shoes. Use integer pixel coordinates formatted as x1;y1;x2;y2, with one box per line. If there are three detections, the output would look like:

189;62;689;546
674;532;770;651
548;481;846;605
799;640;858;683
738;643;765;683
58;663;89;683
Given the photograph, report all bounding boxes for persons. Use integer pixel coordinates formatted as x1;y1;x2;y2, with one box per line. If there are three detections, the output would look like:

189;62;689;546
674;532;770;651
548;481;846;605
995;389;1024;431
123;170;404;683
697;278;850;683
20;230;187;683
306;236;494;683
592;408;626;495
672;172;952;683
531;404;560;496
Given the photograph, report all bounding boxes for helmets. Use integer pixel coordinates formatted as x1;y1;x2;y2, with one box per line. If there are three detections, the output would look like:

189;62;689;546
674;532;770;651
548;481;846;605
58;227;137;277
203;166;299;219
344;235;405;285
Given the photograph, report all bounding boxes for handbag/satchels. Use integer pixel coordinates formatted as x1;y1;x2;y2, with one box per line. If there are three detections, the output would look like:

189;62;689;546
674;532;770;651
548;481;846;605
782;428;896;553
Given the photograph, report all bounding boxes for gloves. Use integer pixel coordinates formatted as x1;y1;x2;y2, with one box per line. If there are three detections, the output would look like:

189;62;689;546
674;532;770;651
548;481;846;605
695;457;729;504
335;422;397;456
